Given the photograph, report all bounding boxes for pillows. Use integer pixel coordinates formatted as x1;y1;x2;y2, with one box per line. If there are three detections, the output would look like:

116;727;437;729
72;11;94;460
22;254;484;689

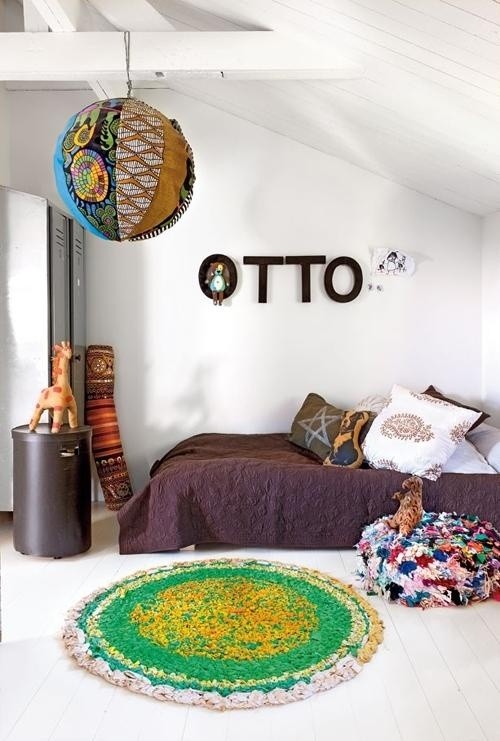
287;384;492;481
84;343;134;512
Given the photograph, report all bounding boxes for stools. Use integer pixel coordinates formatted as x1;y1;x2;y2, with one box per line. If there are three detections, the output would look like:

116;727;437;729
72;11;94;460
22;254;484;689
355;510;500;609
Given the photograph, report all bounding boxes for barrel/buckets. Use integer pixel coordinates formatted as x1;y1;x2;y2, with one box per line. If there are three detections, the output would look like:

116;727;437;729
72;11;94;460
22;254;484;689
12;422;93;557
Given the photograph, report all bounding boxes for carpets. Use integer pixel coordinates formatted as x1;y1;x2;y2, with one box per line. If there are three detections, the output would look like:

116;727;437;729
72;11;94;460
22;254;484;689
61;556;385;711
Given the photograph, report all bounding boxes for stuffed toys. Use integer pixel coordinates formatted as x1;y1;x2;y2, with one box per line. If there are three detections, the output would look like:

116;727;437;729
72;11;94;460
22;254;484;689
27;341;79;433
204;262;230;307
384;476;424;538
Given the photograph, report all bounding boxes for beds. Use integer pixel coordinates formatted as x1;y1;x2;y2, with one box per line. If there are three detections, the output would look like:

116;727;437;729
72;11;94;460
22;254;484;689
116;422;500;556
